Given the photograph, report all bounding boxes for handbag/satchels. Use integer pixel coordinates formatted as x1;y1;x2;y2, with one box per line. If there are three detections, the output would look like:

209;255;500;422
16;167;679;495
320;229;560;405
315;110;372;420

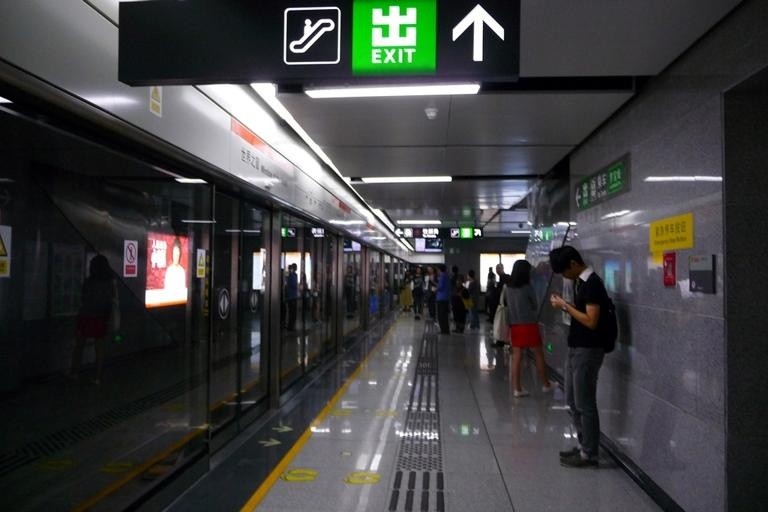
492;284;512;345
462;292;474;309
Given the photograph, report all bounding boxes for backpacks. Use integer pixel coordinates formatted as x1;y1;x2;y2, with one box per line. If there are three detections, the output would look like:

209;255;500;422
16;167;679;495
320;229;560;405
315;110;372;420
601;295;617;353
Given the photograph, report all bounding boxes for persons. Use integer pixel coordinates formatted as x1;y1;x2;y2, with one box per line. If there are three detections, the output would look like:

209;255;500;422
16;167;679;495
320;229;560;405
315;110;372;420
78;251;124;391
161;238;187;303
502;260;561;399
401;263;511;347
547;246;617;469
282;260;324;331
346;262;359;320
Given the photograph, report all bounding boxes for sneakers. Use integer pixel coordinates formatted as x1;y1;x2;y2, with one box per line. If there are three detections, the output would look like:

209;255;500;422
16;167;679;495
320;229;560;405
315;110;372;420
437;328;480;335
513;389;529;397
542;381;560;392
414;315;421;321
559;447;599;470
